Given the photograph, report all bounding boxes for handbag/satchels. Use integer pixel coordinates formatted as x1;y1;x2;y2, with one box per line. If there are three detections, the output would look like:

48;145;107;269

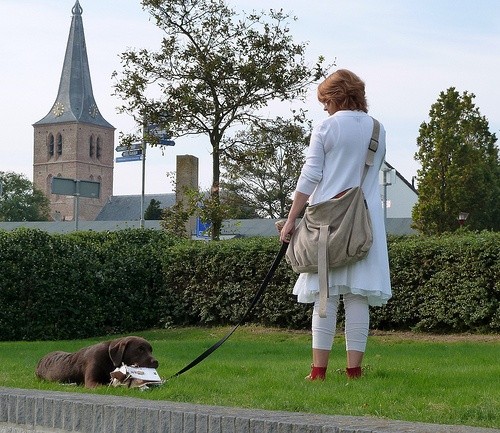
285;188;373;273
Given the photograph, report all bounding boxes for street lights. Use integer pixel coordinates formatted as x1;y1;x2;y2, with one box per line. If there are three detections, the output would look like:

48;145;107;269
456;211;469;231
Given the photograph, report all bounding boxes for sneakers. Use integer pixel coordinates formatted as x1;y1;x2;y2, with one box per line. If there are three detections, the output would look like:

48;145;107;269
304;368;327;381
347;367;361;382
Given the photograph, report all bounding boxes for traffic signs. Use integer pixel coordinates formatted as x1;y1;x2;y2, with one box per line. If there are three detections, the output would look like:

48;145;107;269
114;123;175;163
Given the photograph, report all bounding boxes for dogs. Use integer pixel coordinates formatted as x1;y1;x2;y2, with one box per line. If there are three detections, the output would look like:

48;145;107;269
34;336;159;389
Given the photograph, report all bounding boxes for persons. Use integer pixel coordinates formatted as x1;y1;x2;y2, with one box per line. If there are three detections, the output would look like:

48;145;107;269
280;69;392;381
